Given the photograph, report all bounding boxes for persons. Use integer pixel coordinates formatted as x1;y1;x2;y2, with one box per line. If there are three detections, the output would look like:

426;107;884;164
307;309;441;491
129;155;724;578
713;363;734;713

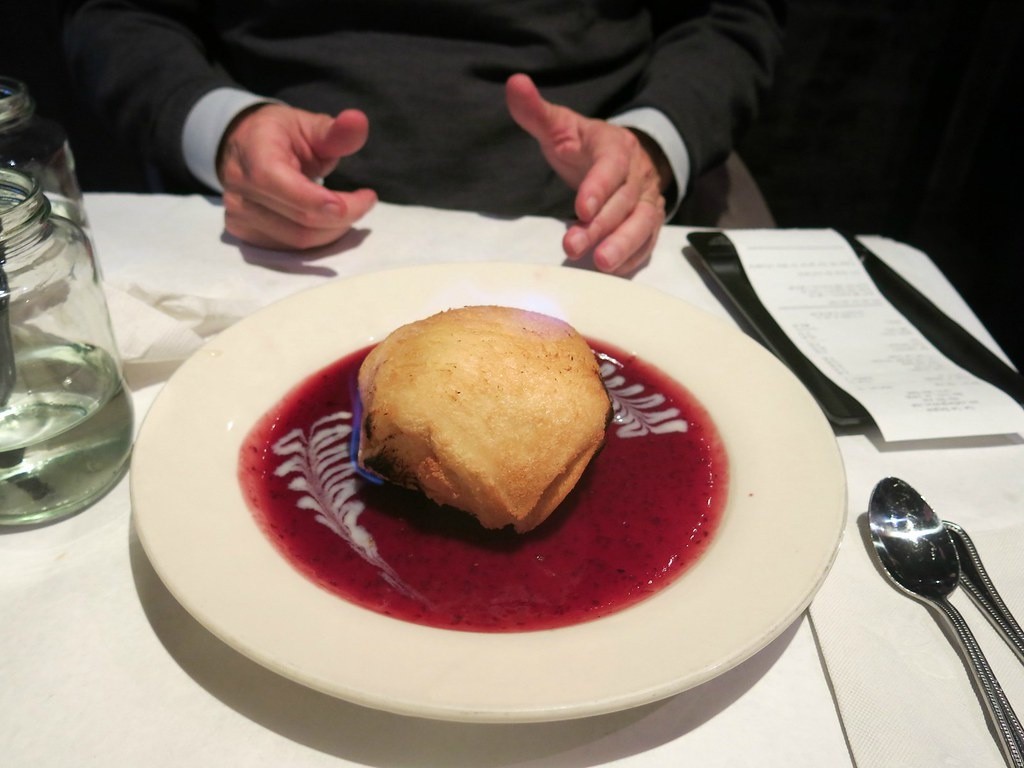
60;0;784;277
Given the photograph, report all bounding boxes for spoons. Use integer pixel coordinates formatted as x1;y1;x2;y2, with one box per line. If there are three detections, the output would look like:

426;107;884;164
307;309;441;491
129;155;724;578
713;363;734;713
867;475;1024;768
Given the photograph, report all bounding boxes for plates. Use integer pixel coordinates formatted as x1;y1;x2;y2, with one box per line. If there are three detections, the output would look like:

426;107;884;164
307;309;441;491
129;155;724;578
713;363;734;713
128;262;849;726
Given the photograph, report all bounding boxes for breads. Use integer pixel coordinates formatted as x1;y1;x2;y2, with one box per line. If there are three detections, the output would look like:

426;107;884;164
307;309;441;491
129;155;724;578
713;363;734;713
357;306;610;531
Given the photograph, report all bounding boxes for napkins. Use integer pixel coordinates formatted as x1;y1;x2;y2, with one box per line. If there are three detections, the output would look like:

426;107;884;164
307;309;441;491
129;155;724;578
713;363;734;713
22;255;241;365
0;193;1024;768
807;527;1024;768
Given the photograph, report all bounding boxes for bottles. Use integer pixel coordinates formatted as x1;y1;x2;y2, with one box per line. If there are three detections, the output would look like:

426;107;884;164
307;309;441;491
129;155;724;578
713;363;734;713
0;75;102;282
1;167;134;529
